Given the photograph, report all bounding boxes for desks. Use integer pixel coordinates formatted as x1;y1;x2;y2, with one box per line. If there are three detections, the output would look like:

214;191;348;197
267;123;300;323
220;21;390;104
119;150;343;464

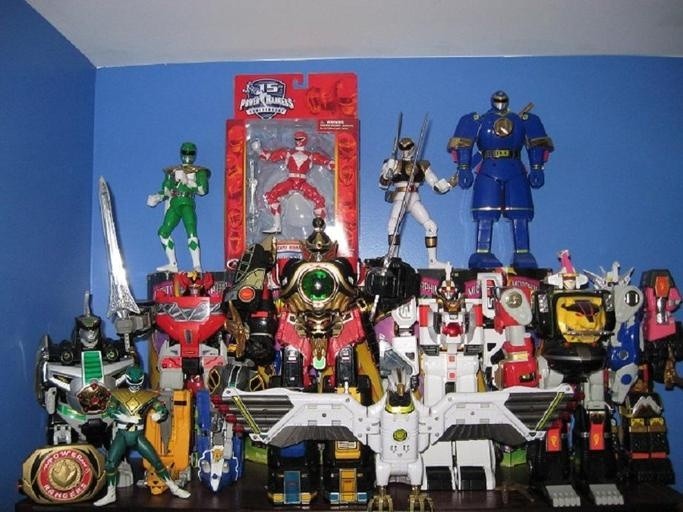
12;456;682;512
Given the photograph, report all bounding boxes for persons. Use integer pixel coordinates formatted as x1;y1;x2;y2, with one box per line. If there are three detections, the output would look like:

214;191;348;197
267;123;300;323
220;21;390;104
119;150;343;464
19;88;683;512
